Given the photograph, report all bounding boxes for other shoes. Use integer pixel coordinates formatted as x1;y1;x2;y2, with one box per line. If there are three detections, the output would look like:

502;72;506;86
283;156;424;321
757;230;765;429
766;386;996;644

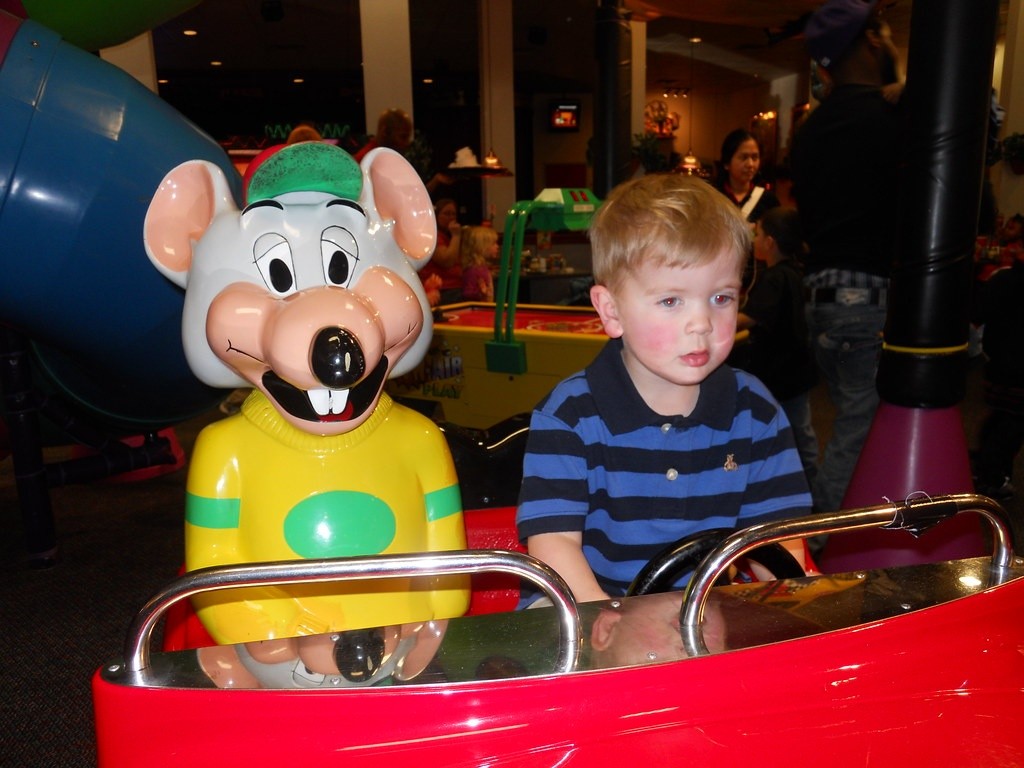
976;478;1018;501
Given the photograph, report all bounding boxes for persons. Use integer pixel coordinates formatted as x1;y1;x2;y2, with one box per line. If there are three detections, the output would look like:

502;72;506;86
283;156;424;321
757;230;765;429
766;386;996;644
590;591;727;670
719;1;1023;554
418;200;499;305
515;175;813;610
352;107;453;191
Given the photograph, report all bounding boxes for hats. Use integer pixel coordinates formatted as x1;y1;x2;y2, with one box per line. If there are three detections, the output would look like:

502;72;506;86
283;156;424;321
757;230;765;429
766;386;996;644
806;0;884;71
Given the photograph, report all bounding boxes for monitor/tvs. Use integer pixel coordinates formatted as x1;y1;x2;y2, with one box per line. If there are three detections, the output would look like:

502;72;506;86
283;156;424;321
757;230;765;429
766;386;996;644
545;104;580;134
